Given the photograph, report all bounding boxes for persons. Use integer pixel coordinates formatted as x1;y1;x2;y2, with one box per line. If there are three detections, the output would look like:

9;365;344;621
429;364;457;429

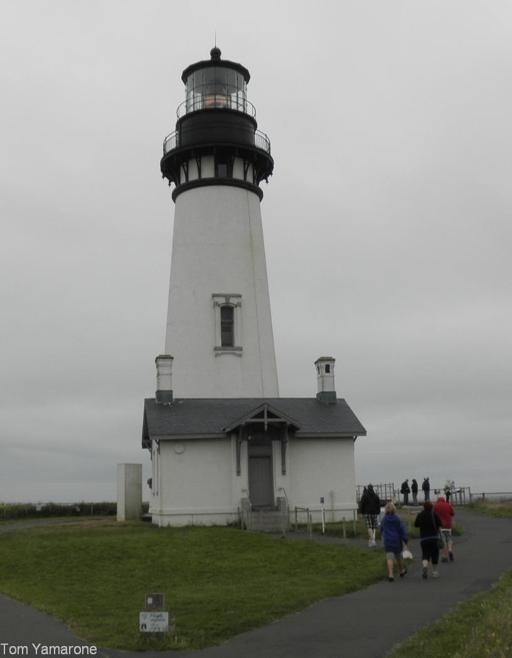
422;478;431;502
400;479;410;504
378;503;409;582
413;502;443;579
411;478;418;505
433;497;454;563
360;483;381;548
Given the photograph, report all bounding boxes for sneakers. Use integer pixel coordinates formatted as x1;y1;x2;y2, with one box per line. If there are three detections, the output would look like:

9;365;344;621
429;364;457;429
441;556;448;563
448;551;455;561
368;535;376;548
432;571;440;579
422;567;428;580
389;577;394;582
399;570;408;577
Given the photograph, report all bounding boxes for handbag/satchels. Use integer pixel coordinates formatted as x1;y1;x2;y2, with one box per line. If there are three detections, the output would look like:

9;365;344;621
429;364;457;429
401;546;413;560
439;535;445;549
